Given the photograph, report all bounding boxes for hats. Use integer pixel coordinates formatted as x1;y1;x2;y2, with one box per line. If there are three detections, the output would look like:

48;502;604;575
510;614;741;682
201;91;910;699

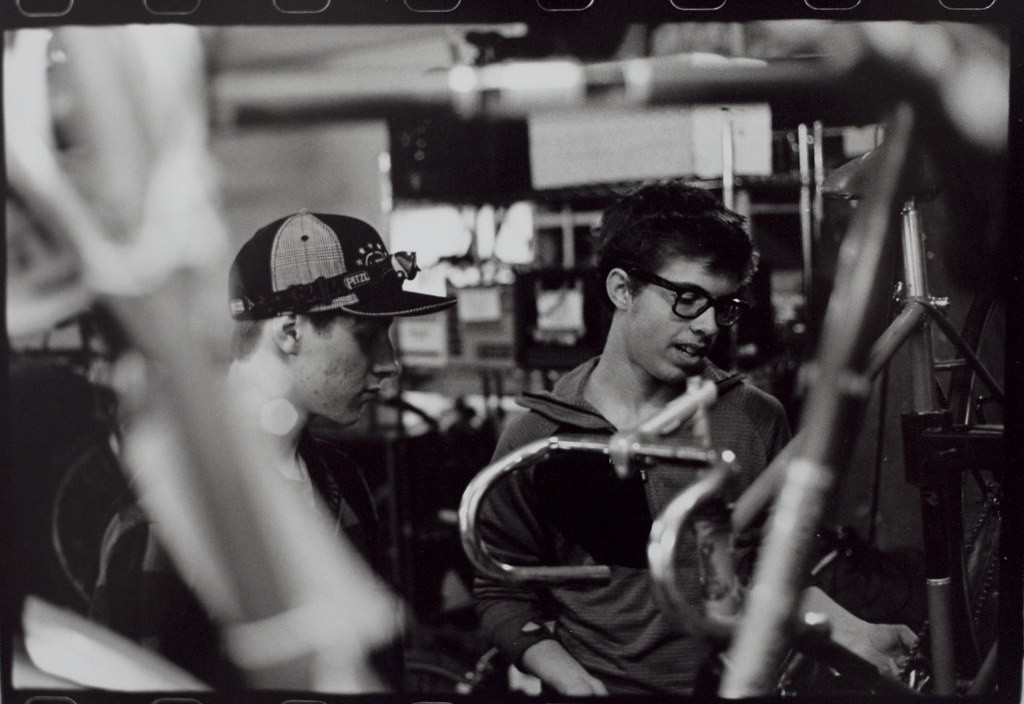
228;211;460;318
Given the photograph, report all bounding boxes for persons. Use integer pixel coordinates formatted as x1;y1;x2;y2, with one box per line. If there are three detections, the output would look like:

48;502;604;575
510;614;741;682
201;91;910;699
472;181;920;698
93;211;449;693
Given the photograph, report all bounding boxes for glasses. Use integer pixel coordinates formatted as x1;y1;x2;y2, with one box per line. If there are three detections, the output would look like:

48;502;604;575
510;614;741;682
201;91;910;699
629;265;751;328
